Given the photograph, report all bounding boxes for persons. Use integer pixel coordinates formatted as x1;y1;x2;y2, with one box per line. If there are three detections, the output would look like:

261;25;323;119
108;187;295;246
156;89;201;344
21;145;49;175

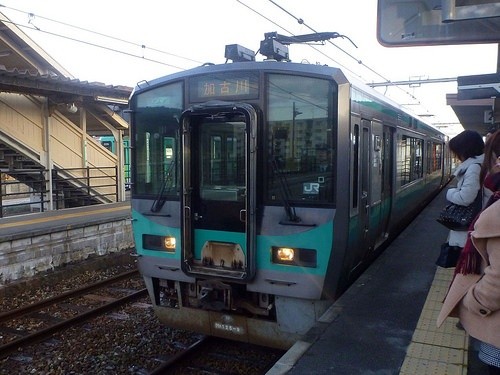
447;129;487;331
437;127;500;375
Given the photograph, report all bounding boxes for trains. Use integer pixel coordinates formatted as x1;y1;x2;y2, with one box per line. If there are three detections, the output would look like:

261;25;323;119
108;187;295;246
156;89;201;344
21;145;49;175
127;31;459;349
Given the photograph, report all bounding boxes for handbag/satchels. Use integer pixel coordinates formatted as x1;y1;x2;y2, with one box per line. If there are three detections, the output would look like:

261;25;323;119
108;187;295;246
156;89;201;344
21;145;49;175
435;242;462;268
436;163;482;231
